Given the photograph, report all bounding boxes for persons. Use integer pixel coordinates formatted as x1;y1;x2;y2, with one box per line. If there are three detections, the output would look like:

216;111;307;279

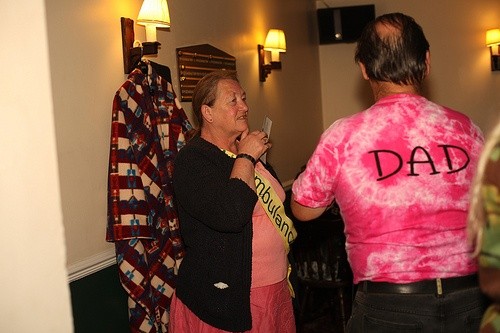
469;122;500;333
168;69;296;333
290;12;487;333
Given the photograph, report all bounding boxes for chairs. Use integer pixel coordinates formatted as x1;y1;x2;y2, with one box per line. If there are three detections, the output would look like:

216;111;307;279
283;188;346;333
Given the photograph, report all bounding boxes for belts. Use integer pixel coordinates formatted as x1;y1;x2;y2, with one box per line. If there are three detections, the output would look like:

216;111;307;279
356;273;480;295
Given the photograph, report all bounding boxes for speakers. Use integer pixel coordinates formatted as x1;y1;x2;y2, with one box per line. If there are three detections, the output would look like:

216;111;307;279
316;4;375;45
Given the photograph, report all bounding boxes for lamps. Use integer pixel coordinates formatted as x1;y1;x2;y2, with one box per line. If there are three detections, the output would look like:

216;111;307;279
121;0;171;74
486;28;500;72
257;28;287;83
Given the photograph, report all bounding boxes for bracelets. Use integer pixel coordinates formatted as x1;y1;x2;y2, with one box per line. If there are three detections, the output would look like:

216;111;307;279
236;154;257;168
